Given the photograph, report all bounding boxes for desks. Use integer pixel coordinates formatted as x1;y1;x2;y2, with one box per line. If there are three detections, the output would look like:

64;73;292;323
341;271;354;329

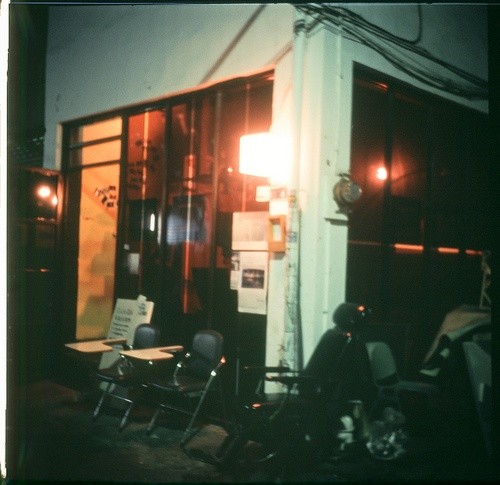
64;338;183;425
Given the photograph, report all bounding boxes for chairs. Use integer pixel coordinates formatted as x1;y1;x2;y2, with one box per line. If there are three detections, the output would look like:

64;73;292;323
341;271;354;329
146;329;241;449
207;297;374;481
90;324;169;433
